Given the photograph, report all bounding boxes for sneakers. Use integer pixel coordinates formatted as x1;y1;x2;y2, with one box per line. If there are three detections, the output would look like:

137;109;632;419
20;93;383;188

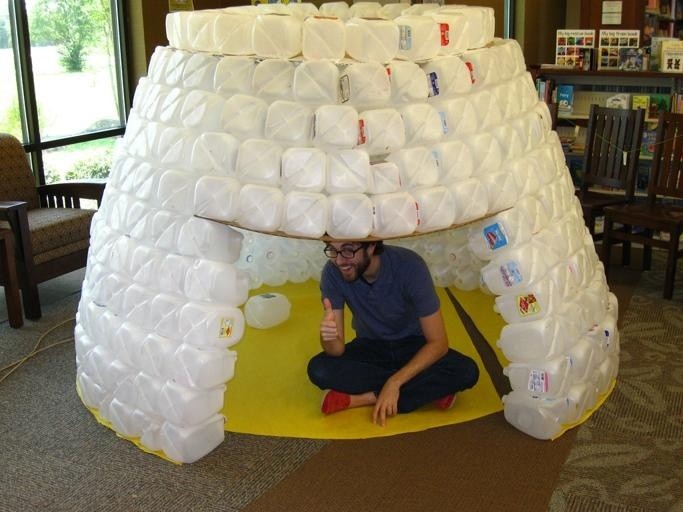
320;390;350;414
432;394;456;412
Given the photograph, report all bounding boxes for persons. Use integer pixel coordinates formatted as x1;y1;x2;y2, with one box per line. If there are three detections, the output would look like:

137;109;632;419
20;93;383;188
306;238;479;425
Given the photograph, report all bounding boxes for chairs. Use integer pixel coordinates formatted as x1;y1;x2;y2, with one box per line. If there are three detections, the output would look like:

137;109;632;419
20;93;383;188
0;133;106;321
576;104;645;267
548;104;558;131
603;110;683;301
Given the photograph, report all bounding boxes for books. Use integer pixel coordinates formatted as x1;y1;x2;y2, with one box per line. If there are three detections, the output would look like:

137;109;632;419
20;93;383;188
533;38;682;160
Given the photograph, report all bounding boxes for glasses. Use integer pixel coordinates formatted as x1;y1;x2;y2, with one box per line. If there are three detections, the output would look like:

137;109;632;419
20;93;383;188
324;243;366;259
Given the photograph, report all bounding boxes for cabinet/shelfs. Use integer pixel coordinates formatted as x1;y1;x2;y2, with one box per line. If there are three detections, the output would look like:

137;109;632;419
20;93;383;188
539;68;683;251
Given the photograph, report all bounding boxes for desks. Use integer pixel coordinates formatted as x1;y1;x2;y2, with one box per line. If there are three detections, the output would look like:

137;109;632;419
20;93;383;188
0;228;24;329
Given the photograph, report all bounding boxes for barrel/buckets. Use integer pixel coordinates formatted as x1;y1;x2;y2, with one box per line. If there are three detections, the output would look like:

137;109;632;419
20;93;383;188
73;1;620;465
